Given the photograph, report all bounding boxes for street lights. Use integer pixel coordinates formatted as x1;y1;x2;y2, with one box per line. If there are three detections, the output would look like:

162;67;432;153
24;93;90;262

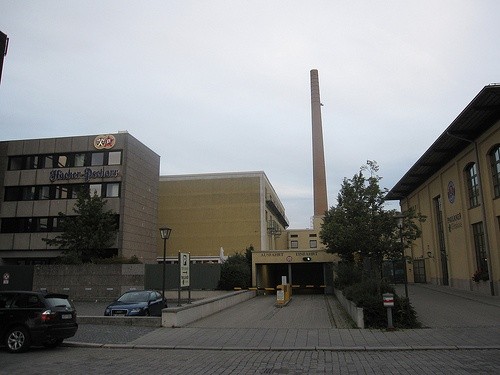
394;212;413;329
159;226;173;315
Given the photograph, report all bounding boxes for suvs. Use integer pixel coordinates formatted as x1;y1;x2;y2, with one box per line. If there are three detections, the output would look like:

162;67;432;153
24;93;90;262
0;290;78;354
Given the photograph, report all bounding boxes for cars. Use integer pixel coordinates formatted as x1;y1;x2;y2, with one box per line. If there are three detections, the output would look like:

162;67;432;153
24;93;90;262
104;289;168;317
